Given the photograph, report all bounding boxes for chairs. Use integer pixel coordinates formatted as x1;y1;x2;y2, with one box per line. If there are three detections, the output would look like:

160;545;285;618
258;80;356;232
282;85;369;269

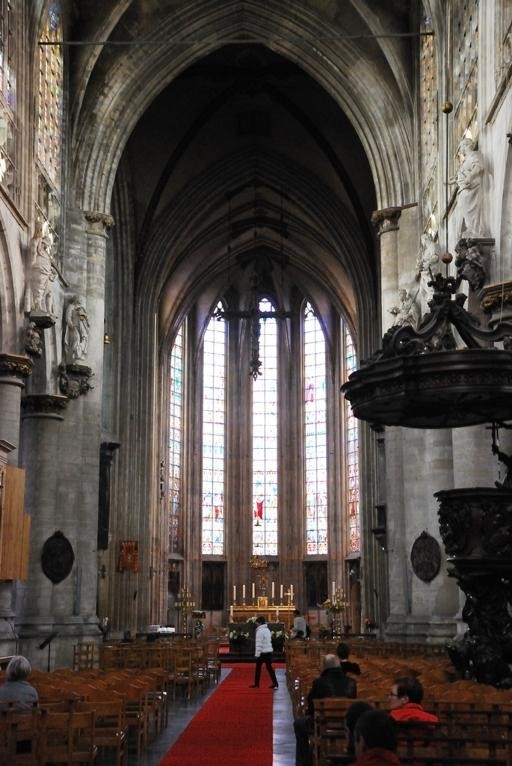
281;639;512;765
1;634;222;765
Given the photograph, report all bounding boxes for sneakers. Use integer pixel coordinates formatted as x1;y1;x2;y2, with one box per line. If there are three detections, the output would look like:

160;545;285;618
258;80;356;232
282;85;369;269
268;685;278;689
249;685;259;688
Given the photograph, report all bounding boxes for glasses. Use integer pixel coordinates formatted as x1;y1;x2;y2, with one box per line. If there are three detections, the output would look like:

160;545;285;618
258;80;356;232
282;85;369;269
391;692;399;696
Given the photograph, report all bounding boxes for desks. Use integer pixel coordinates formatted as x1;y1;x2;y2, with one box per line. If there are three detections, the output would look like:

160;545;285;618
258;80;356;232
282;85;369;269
229;605;296;631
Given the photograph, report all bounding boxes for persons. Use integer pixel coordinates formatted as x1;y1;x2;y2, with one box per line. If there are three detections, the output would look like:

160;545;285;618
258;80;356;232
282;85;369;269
293;644;438;766
291;610;306;638
29;222;57;316
64;295;90;360
99;616;112;640
249;616;279;690
168;563;179;608
388;289;419;329
0;655;39;755
124;624;130;639
195;621;202;639
421;233;439;315
257;590;269;608
457;138;484;237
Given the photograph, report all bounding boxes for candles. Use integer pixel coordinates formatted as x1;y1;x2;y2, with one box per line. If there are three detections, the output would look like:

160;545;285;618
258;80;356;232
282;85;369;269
233;585;237;600
280;585;284;598
242;584;245;598
332;582;336;595
291;584;293;600
252;582;256;599
272;582;275;599
